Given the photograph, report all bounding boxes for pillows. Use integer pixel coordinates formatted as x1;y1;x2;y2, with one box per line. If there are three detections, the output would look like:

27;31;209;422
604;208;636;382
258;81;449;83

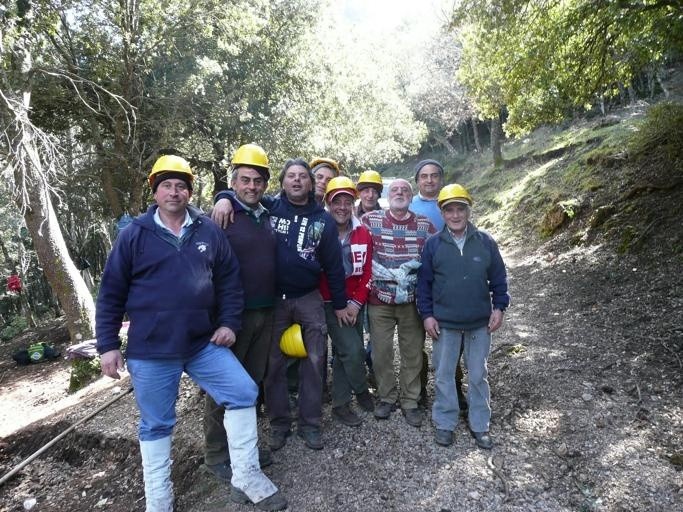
149;155;193;188
231;144;270;168
280;323;307;358
438;184;472;209
309;158;383;203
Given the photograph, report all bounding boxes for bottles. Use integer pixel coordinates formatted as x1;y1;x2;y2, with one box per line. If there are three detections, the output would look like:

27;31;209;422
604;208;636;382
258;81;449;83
415;159;444;182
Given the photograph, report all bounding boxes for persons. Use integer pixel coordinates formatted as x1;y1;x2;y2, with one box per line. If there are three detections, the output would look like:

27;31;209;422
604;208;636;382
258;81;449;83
314;174;375;427
304;155;341;209
209;157;352;451
357;177;440;429
94;150;290;512
415;183;510;449
405;159;470;411
200;142;283;483
328;169;386;389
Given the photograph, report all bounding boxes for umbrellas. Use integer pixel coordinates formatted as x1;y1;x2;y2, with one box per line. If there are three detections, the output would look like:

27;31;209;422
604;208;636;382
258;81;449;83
267;389;493;450
206;449;287;510
